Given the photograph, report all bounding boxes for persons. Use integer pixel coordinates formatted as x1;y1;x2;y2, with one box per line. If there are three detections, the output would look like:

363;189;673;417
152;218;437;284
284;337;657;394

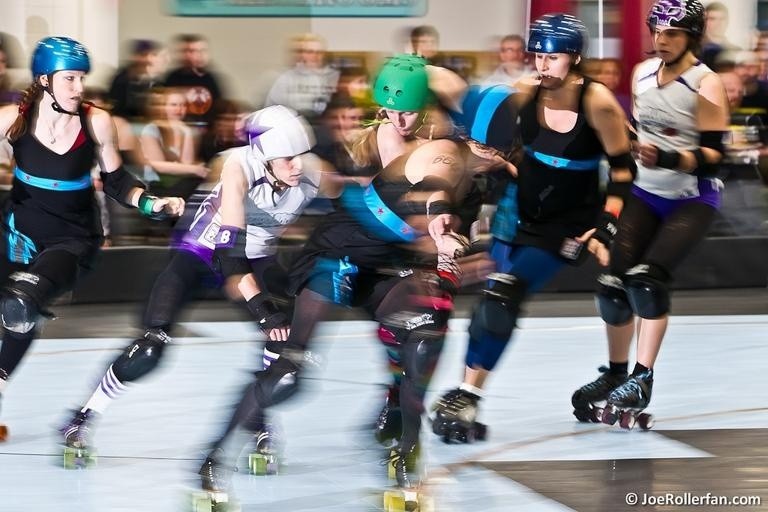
1;38;186;431
3;0;767;203
571;0;728;409
56;105;375;453
198;84;521;493
318;51;455;446
428;12;638;420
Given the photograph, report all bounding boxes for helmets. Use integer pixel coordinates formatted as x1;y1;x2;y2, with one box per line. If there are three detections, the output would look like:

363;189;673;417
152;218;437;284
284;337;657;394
30;37;90;77
464;85;527;151
249;105;317;162
373;54;428;111
646;0;706;36
527;15;589;55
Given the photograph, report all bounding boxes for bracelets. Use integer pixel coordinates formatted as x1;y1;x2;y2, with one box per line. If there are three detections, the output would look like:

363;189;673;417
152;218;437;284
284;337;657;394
139;191;157;216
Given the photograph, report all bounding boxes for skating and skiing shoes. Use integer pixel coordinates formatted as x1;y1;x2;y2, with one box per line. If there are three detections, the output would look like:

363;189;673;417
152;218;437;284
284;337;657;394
64;409;104;471
247;418;285;475
375;382;432;512
194;459;239;511
429;388;486;444
572;368;653;431
